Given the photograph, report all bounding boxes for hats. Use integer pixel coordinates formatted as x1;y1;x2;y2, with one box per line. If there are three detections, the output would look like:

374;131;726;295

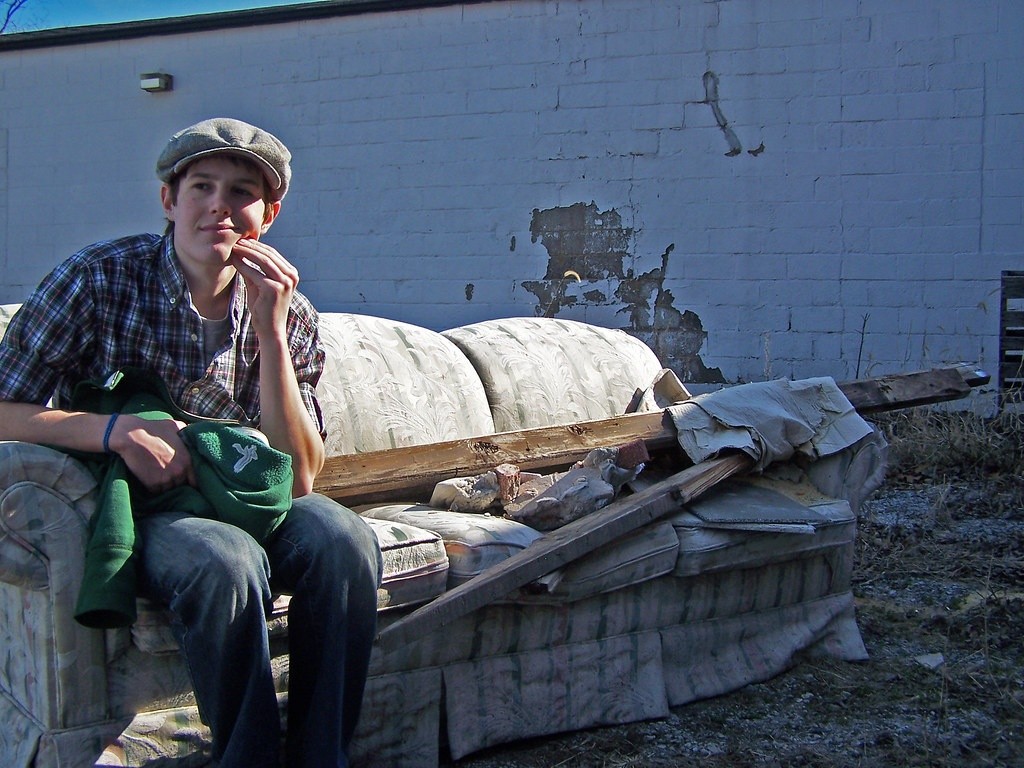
155;118;292;202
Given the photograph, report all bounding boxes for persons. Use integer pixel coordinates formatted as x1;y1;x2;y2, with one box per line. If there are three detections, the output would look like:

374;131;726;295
0;117;385;768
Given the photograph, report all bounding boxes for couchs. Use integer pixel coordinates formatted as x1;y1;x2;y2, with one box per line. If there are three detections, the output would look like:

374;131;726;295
0;303;890;768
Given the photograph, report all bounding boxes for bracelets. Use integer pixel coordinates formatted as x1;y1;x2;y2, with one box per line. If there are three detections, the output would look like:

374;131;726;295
101;412;123;451
